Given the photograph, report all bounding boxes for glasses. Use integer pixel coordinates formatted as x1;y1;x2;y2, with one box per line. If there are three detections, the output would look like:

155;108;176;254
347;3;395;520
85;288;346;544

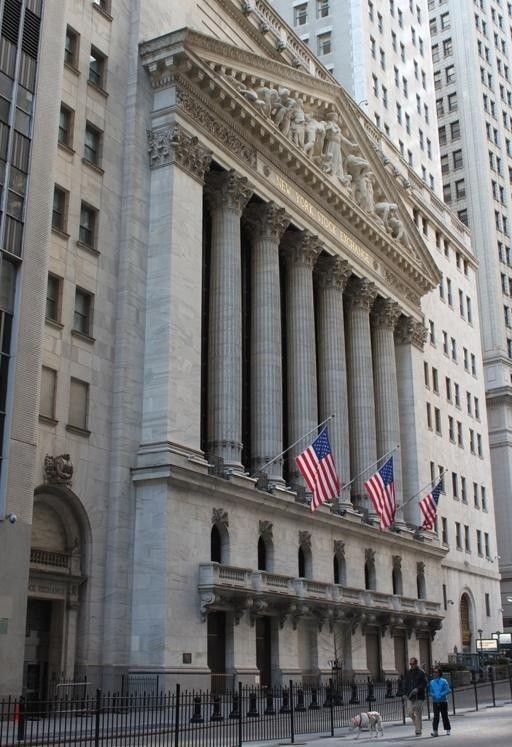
410;663;415;665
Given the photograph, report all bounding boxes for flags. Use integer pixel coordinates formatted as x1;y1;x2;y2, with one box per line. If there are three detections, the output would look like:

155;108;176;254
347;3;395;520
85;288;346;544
294;424;341;513
418;478;444;531
363;454;397;533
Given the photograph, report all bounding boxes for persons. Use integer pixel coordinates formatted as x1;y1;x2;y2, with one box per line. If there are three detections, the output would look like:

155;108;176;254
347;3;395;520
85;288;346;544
429;666;451;737
401;658;427;736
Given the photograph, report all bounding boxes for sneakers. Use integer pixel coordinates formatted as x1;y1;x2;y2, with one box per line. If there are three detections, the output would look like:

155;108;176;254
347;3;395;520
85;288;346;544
447;730;451;735
415;730;422;736
430;731;438;737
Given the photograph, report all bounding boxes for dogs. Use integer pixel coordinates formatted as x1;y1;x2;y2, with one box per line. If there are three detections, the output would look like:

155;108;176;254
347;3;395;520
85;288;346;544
348;711;384;740
